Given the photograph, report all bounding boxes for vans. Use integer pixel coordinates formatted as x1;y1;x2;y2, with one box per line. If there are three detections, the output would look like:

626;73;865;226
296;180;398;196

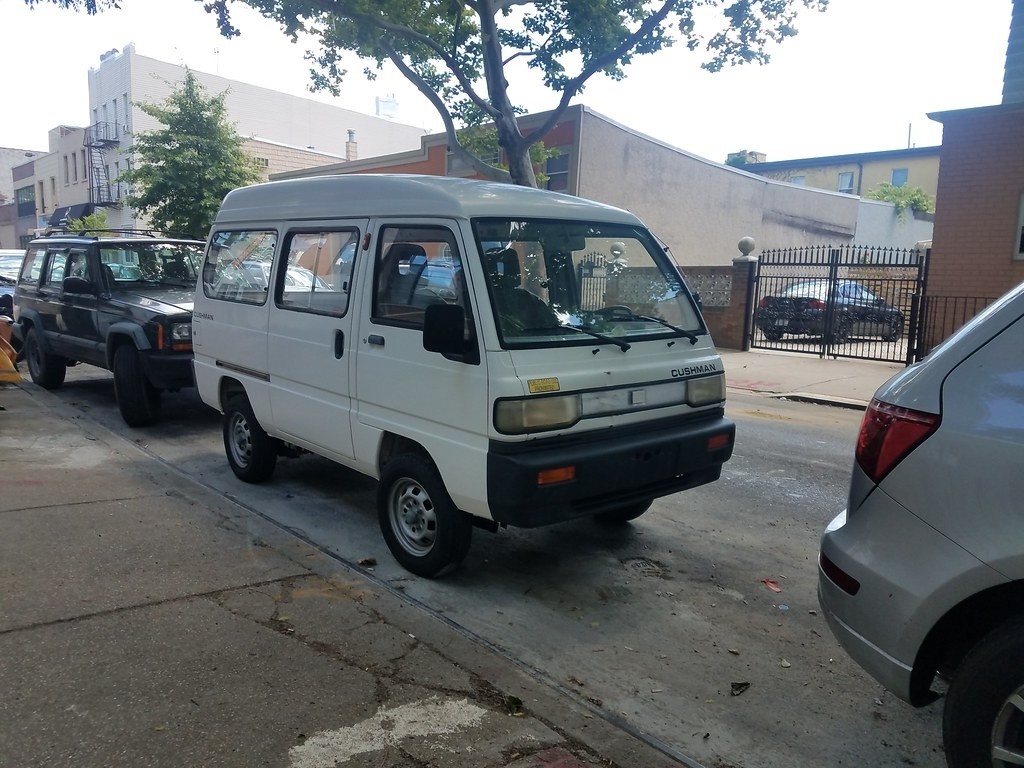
192;173;735;579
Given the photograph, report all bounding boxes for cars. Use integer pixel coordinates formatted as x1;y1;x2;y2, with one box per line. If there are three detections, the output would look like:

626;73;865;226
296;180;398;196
755;278;906;344
816;279;1024;768
0;249;84;316
104;262;143;282
392;257;463;302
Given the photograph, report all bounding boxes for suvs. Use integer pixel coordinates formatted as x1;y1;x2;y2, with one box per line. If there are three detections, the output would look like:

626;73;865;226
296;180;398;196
11;229;267;430
240;261;334;293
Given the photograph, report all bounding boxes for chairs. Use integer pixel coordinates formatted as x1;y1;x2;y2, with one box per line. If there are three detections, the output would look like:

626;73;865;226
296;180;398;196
471;248;561;335
84;262;114;285
374;245;430;319
164;259;192;279
